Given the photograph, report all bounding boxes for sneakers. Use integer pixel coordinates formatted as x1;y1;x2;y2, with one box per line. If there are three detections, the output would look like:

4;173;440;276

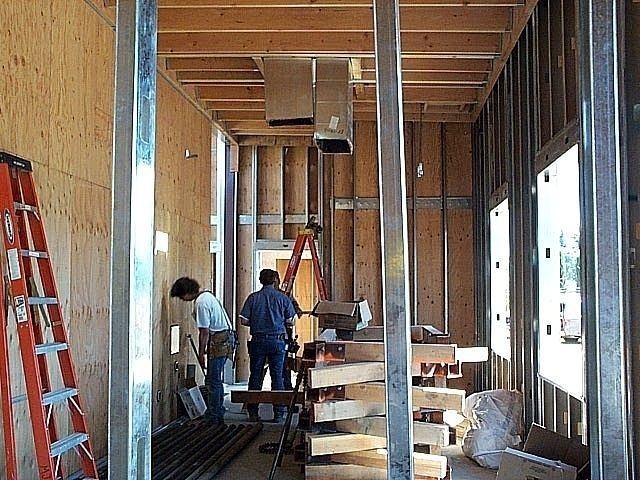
249;413;260;422
273;413;284;423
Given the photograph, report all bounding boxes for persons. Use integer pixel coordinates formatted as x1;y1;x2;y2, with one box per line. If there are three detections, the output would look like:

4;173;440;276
238;269;295;425
272;271;303;414
170;276;233;424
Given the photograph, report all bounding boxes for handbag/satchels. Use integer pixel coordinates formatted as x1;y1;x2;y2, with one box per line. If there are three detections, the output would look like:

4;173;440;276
207;331;235;359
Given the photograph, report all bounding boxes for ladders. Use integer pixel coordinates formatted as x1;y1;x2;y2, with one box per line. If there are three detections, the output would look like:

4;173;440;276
0;152;99;480
241;229;329;413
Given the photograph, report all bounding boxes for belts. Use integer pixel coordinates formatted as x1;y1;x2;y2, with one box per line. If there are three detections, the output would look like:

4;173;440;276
252;332;287;338
